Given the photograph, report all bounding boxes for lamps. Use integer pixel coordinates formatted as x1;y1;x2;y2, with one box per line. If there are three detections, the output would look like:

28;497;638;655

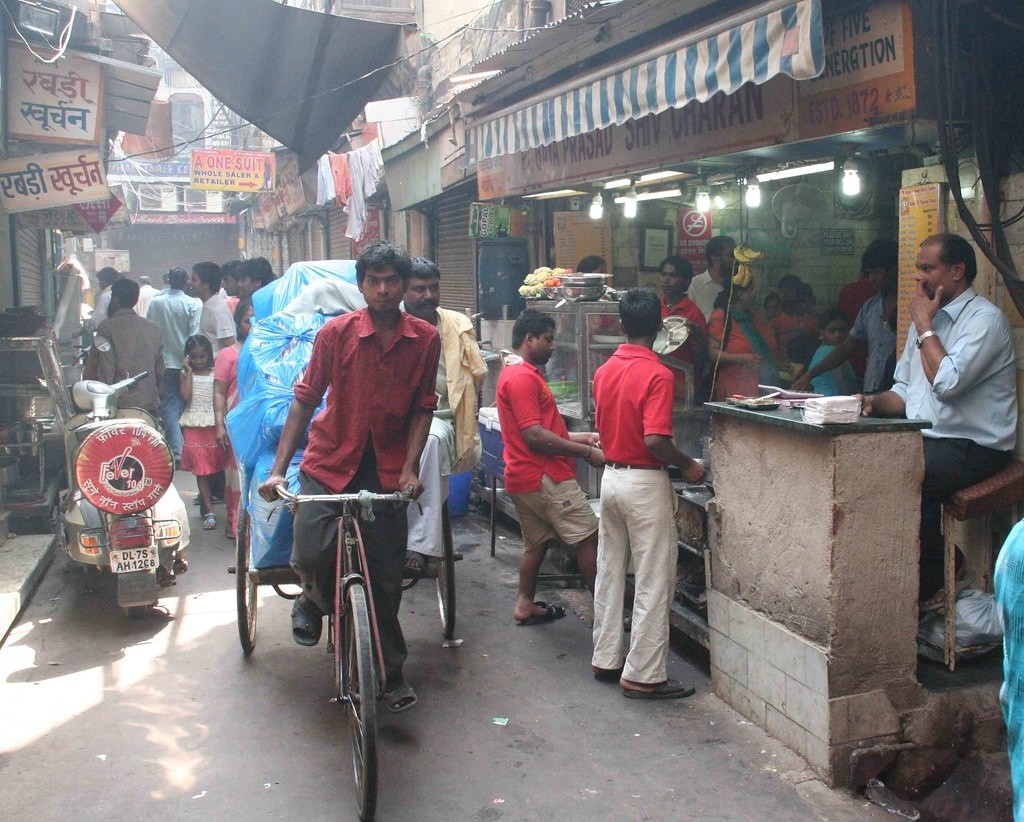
592;168;685;191
714;182;742;210
6;0;61;42
754;157;838;184
613;180;689;204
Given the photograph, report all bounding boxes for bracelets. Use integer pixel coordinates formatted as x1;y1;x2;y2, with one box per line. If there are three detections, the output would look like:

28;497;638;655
585;445;592;462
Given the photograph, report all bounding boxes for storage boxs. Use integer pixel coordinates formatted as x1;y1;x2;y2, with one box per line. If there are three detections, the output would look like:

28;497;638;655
472;404;506;482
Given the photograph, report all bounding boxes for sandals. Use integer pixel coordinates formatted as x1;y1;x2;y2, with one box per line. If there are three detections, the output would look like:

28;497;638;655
202;513;216;530
404;551;426;571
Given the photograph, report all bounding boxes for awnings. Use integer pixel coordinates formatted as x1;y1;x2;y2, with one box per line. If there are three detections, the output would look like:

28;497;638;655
470;0;626;75
99;11;156;67
29;40;164;136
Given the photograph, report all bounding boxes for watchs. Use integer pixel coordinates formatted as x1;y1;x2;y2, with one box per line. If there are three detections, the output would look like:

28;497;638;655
916;330;939;349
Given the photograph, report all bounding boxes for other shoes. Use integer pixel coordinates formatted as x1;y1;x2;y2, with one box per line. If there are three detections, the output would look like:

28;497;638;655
224;521;235;538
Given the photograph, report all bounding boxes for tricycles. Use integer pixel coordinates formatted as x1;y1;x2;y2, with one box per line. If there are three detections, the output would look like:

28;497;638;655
228;259;463;822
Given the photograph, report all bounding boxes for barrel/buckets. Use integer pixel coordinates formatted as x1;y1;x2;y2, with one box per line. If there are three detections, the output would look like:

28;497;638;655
448;471;474;518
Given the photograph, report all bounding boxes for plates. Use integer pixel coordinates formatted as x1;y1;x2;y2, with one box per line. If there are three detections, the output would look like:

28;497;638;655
592;334;627;344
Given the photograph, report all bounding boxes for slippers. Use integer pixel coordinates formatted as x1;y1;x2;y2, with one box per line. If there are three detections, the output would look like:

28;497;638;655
918;569;975;612
592;659;626;680
291;593;323;646
381;678;417;713
623;678;696;699
521;601;566;625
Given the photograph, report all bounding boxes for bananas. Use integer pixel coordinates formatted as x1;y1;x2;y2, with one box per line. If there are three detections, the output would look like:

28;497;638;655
732;243;765;287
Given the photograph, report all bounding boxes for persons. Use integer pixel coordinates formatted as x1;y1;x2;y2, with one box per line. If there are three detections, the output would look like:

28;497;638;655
653;235;900;404
259;241;440;712
861;233;1017;612
556;256;619;382
993;514;1024;822
406;255;489;570
497;308;633;631
592;289;707;699
83;256;278;574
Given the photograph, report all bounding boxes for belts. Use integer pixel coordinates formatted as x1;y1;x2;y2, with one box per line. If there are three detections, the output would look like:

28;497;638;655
606;461;668;471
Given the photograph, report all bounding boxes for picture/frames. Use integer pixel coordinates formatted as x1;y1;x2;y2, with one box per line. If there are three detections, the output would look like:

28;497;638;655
638;223;673;273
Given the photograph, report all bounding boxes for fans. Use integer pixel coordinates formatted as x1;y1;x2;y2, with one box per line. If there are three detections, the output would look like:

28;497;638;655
771;175;832;237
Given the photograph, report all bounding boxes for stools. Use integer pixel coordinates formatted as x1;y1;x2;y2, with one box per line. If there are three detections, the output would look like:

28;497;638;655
933;461;1024;677
0;437;47;508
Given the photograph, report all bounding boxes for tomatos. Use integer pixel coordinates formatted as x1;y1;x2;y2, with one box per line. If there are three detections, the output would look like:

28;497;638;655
545;269;573;287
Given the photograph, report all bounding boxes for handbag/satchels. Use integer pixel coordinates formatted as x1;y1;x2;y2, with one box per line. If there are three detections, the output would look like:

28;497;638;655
224;260;404;567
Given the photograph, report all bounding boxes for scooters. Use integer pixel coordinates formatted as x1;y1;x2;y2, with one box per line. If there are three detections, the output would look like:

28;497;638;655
56;370;191;620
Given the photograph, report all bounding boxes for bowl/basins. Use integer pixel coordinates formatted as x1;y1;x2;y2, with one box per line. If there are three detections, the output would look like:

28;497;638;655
554;273;614;286
608;291;628;301
557;285;607;302
542;287;564;300
